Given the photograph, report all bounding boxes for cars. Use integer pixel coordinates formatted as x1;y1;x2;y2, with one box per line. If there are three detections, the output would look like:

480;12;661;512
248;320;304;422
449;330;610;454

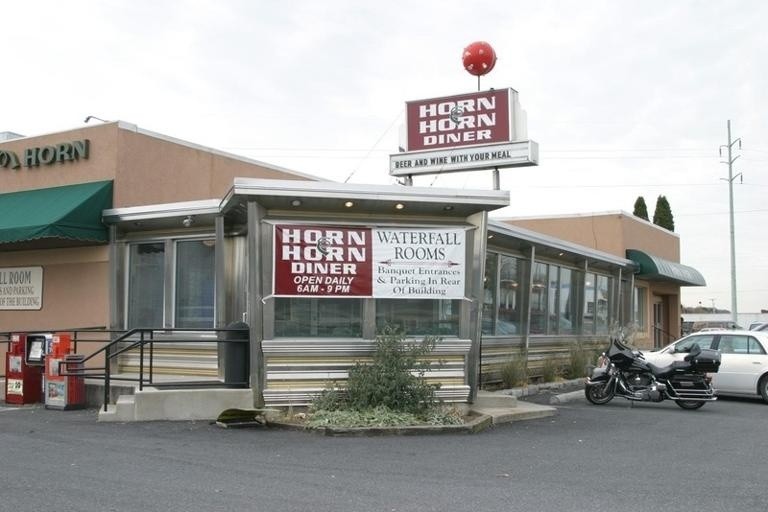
628;328;768;402
483;276;544;292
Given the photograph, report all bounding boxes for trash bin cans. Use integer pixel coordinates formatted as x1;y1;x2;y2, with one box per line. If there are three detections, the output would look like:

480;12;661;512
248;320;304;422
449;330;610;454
217;321;250;384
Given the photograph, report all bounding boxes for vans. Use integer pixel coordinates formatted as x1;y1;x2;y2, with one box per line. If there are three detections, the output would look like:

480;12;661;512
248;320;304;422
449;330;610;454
489;308;592;335
691;318;768;331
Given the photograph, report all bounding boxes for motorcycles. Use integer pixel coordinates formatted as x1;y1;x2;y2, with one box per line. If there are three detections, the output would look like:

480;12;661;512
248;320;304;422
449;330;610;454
584;332;722;410
